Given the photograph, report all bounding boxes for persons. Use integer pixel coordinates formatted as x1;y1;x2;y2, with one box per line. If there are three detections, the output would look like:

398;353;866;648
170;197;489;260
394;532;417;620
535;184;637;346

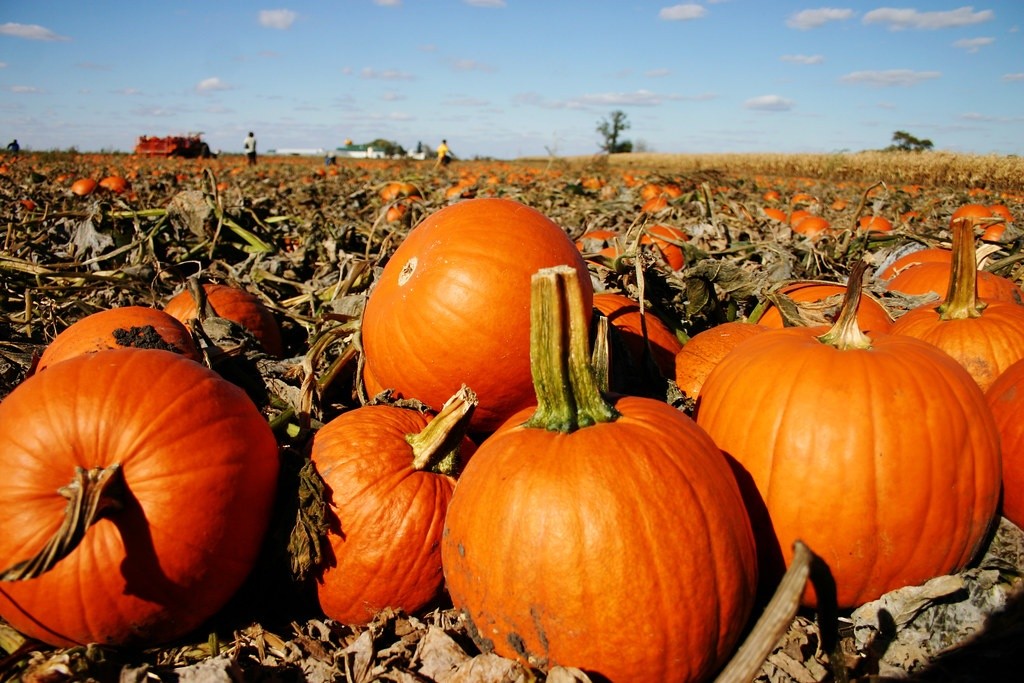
325;149;337;166
433;139;455;169
8;139;19;157
244;132;257;164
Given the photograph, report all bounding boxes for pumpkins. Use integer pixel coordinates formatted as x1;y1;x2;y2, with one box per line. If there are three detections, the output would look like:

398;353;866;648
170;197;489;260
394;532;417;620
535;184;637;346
259;200;1024;683
0;284;292;654
0;136;1024;271
